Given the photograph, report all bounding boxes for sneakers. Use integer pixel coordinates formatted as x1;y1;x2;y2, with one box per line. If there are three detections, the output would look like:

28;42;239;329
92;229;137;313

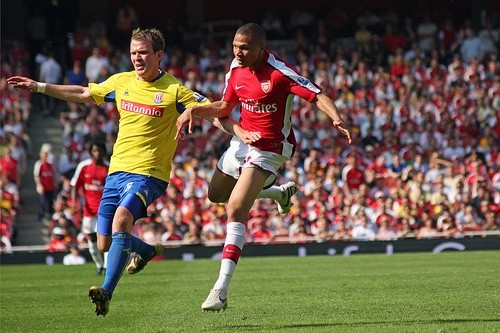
275;180;298;214
201;289;227;312
126;243;164;274
89;286;110;317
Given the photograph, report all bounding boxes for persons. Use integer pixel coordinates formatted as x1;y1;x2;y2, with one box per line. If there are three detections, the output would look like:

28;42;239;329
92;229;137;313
0;32;35;253
34;141;63;224
171;21;351;313
5;29;262;318
70;141;110;275
39;52;63;116
46;7;500;247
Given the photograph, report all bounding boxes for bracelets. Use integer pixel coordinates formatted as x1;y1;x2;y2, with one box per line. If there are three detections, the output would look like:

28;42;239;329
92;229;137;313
35;82;47;93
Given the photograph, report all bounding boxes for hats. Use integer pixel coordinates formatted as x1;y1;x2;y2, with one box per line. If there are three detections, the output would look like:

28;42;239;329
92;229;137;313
69;243;78;249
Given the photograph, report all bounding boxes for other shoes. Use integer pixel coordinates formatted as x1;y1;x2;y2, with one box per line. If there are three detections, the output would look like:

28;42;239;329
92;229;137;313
97;266;106;275
